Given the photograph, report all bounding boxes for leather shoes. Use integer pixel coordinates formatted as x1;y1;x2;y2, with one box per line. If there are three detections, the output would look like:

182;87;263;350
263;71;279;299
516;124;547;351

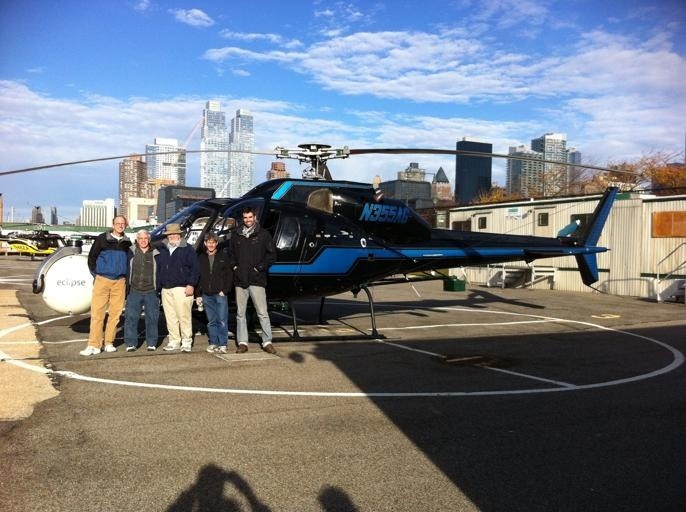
236;344;248;353
263;344;276;353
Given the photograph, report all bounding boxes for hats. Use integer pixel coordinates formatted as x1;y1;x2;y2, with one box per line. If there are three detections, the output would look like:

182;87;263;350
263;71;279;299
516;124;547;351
164;224;183;235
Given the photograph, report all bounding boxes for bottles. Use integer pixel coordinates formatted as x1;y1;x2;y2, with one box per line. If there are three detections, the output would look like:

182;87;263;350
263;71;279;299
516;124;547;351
198;300;204;312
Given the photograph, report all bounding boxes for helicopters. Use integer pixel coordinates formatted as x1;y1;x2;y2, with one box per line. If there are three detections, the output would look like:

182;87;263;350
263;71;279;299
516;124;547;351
1;223;67;261
1;144;643;343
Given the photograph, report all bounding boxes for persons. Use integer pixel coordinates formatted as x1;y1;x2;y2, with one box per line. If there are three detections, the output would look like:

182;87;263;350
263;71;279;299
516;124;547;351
78;215;131;357
228;206;278;355
124;229;160;352
155;223;200;353
195;231;234;354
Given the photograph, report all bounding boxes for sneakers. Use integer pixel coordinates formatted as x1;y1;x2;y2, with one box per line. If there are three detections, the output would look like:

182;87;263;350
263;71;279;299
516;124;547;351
80;343;191;356
206;345;227;353
